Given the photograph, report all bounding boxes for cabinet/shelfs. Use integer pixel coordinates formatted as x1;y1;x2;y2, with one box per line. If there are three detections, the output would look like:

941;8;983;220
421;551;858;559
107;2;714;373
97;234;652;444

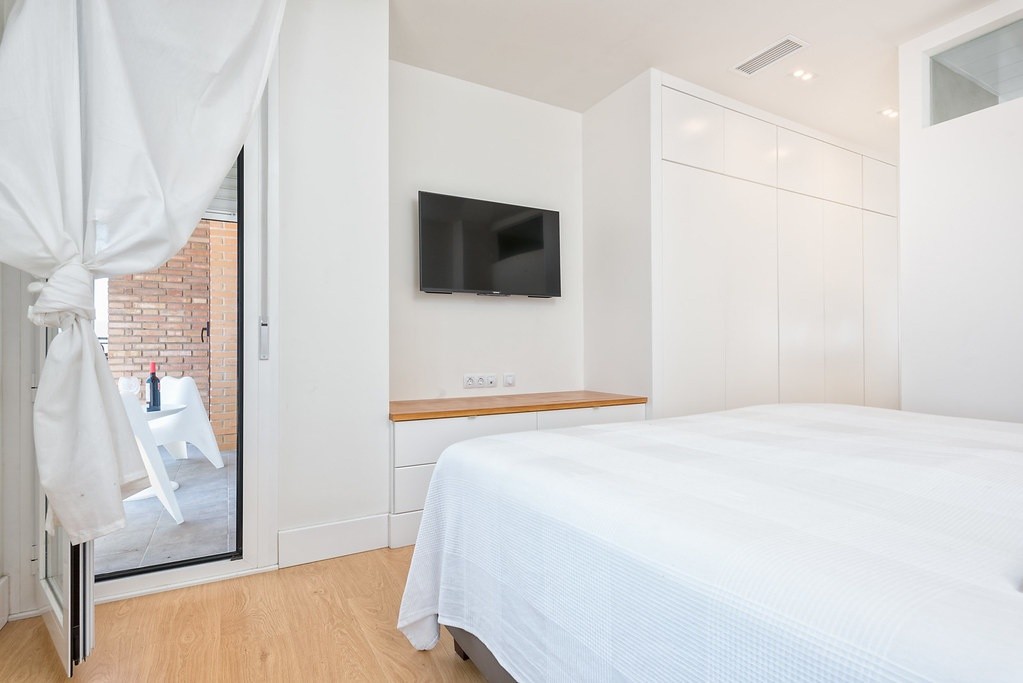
389;390;647;515
580;69;902;421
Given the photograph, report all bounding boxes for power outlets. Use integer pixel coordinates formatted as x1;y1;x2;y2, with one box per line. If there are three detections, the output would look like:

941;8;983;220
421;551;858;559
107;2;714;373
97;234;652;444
463;374;497;388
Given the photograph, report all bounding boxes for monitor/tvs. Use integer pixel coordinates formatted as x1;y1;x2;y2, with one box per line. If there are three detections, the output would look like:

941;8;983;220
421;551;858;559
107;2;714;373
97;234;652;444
418;191;562;298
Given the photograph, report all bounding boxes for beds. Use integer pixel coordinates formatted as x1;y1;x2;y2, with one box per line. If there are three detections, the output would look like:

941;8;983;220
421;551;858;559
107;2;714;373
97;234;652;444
392;399;1023;682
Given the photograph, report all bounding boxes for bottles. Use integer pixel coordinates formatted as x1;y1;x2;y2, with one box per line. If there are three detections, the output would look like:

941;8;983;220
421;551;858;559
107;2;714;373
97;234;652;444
145;361;161;411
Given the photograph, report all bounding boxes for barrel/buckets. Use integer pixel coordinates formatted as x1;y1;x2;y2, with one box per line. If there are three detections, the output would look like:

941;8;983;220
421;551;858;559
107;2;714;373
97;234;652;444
119;376;141;401
